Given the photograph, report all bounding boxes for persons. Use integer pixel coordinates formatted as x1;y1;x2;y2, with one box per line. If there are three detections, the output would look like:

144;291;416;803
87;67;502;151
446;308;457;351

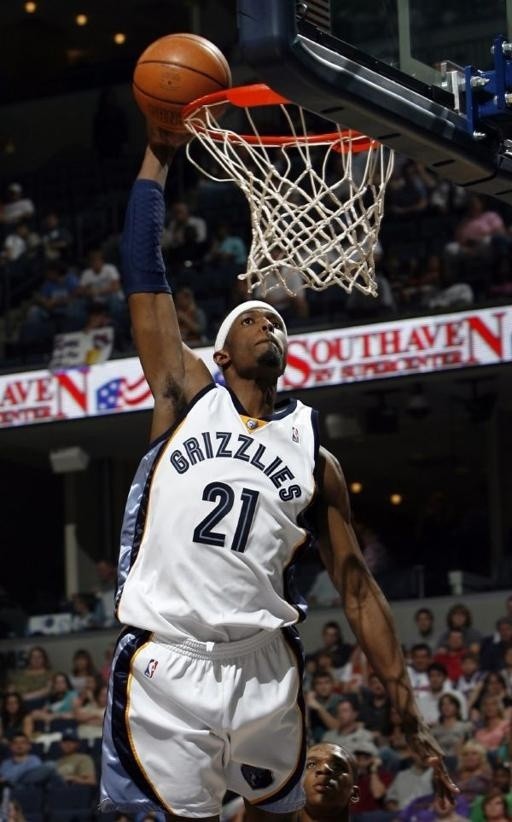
1;89;512;821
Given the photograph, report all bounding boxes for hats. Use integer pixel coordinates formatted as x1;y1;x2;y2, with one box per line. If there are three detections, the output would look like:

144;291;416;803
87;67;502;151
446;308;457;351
61;728;78;741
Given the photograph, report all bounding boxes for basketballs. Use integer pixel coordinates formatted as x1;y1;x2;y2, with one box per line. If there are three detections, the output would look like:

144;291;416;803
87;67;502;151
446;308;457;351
132;31;234;133
